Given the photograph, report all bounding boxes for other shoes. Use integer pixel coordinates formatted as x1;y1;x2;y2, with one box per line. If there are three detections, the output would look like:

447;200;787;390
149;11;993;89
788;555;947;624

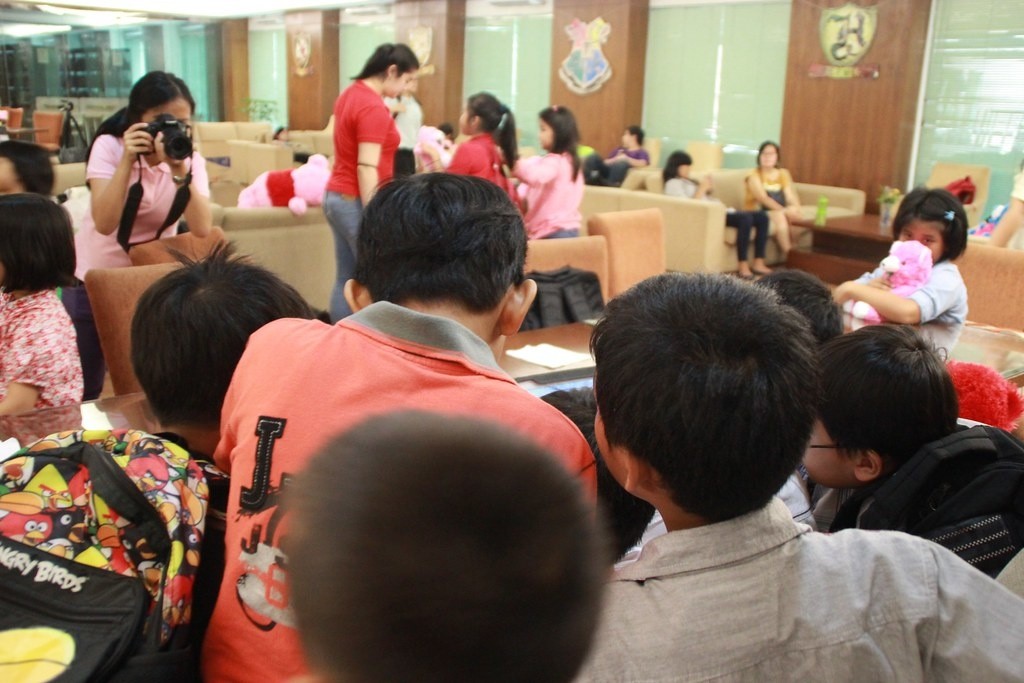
738;267;754;280
751;267;772;275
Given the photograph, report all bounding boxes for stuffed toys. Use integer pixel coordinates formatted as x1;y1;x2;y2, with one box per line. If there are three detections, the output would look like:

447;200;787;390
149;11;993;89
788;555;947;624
414;125;450;172
238;155;330;214
517;156;540;199
844;240;933;322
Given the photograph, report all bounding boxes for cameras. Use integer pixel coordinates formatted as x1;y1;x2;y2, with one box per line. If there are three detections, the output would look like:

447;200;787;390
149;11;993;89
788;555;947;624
137;113;193;161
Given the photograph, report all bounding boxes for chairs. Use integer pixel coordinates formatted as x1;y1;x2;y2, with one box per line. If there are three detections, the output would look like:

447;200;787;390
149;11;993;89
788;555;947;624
31;113;63;152
0;106;25;140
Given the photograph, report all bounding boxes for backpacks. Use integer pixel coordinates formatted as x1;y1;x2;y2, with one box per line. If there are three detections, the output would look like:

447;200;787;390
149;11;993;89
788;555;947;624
517;264;606;336
853;423;1024;581
0;427;234;683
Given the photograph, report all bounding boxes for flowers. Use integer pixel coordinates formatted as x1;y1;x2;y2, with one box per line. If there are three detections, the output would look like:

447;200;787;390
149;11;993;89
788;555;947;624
877;183;903;203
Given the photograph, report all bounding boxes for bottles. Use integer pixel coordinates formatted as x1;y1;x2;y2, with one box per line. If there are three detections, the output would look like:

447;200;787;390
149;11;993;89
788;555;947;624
814;191;828;226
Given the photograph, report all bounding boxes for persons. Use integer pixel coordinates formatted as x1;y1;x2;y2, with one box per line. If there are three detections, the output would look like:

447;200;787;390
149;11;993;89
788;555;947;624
743;141;801;252
285;407;605;683
422;92;518;194
497;106;585;240
1;258;315;683
574;272;1024;683
989;170;1024;250
440;124;452;139
833;188;968;325
201;172;595;682
62;71;211;402
323;43;420;323
0;140;83;418
583;125;649;186
763;270;1024;581
384;76;423;177
664;151;772;279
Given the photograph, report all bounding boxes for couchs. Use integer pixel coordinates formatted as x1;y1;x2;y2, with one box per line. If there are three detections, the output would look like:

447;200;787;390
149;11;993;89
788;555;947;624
51;121;1024;443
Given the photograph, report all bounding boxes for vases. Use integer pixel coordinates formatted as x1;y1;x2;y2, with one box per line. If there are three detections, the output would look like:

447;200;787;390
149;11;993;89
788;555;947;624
878;200;896;227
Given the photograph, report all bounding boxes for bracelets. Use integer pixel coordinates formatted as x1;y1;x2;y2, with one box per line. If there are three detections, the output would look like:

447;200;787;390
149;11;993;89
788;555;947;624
173;175;185;183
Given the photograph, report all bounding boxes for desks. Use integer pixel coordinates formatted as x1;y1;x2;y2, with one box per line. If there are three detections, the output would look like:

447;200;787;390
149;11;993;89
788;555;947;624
498;312;599;396
1;391;164;449
8;128;49;136
781;214;909;275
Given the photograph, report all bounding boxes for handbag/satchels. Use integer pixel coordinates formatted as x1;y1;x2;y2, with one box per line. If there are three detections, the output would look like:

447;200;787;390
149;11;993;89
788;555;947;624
57;115;90;165
942;175;976;205
